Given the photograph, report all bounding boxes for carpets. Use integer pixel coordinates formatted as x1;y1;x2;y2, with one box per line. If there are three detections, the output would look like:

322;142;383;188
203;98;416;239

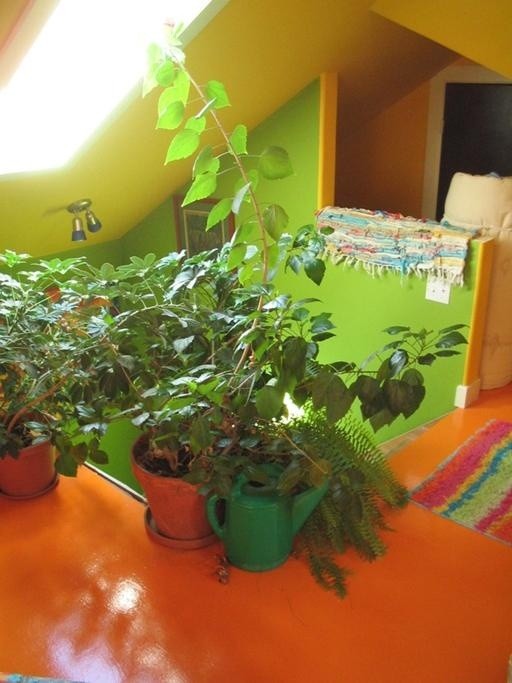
403;420;511;544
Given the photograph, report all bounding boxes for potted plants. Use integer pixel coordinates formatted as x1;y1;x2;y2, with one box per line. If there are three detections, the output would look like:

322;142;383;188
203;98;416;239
132;32;466;551
2;251;225;501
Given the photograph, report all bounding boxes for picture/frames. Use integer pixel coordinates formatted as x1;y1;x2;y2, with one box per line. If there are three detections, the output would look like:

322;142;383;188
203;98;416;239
172;196;233;263
